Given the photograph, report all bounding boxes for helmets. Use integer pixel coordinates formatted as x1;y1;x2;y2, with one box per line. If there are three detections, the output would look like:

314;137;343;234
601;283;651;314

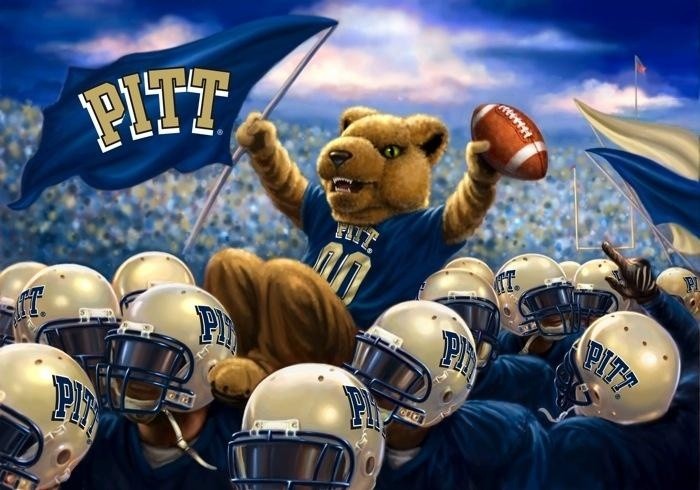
226;362;387;490
664;253;700;320
343;299;477;431
1;333;102;490
0;249;225;345
553;304;683;426
418;252;574;342
104;282;238;422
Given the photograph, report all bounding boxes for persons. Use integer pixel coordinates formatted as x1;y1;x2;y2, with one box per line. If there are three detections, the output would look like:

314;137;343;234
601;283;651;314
0;96;700;490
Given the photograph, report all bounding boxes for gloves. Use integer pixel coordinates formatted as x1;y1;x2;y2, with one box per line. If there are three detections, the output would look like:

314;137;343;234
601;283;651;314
597;238;663;301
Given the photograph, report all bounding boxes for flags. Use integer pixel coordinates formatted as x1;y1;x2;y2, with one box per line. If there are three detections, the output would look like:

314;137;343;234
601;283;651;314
9;16;339;210
636;55;647;75
